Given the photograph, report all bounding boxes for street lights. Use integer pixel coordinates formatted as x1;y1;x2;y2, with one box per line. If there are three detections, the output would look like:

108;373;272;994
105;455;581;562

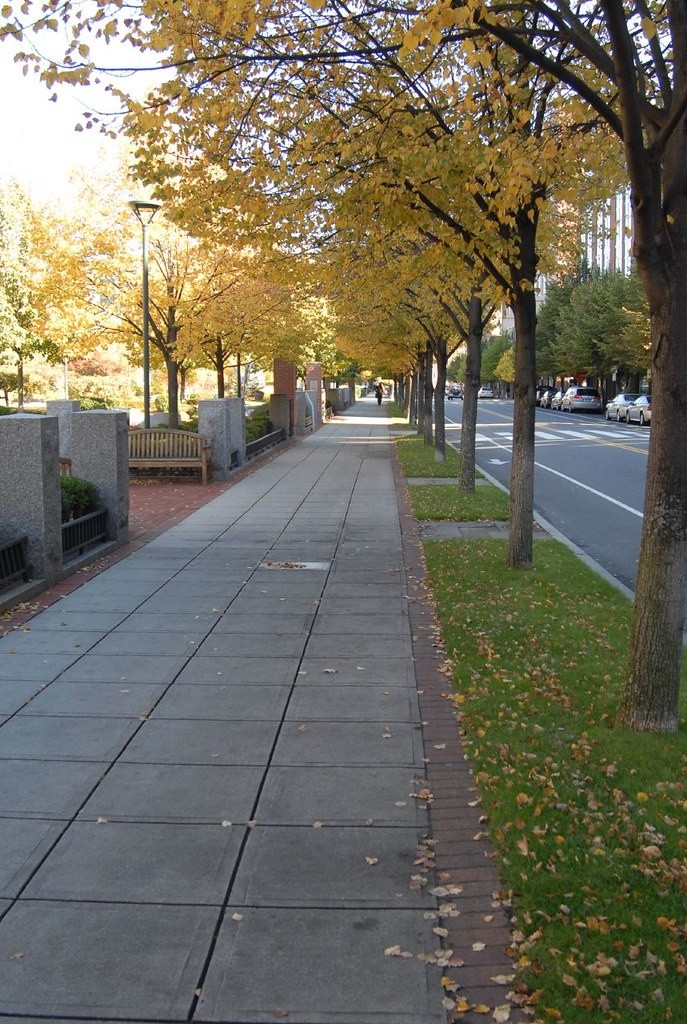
127;201;162;439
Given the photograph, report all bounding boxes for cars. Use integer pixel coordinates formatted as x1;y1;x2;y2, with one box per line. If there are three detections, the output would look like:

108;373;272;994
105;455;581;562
605;394;644;422
539;390;557;409
625;396;652;426
535;385;551;407
479;387;493;398
550;391;566;411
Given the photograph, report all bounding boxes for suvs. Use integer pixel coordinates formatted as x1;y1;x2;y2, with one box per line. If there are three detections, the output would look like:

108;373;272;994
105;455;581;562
561;387;603;414
447;385;463;400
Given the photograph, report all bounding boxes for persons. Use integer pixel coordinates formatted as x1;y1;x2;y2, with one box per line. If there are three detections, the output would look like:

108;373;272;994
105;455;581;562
375;383;383;406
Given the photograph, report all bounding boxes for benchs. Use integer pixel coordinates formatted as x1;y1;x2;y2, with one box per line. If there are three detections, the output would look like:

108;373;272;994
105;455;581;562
128;428;212;486
